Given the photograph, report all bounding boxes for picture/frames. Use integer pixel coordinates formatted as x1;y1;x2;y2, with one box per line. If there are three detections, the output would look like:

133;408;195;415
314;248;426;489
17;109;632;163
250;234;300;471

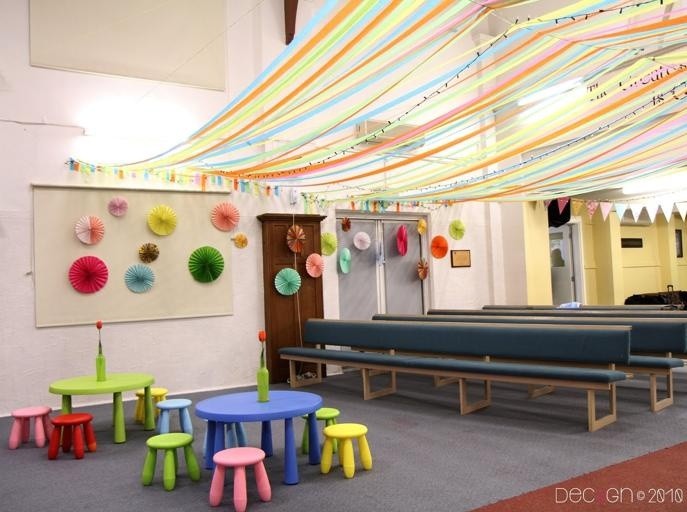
450;249;471;267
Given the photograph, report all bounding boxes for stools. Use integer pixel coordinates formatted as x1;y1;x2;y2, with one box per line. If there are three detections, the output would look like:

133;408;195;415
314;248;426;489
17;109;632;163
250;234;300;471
156;397;194;438
142;433;200;492
135;387;167;424
47;412;97;458
7;406;51;449
301;407;340;453
321;424;373;478
207;446;272;511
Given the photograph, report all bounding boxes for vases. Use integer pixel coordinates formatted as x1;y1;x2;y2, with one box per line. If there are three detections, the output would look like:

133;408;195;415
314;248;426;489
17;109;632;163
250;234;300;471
96;353;104;380
256;368;269;403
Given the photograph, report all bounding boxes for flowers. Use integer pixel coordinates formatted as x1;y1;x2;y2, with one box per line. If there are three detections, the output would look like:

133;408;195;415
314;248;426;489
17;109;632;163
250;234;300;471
96;320;103;355
258;330;266;368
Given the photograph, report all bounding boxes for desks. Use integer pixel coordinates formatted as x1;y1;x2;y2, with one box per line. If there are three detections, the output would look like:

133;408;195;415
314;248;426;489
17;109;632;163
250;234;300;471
49;373;155;445
194;390;323;483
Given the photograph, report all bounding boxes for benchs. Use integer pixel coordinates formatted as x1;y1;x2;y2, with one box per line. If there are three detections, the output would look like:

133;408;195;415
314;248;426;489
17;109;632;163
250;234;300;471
278;300;686;433
